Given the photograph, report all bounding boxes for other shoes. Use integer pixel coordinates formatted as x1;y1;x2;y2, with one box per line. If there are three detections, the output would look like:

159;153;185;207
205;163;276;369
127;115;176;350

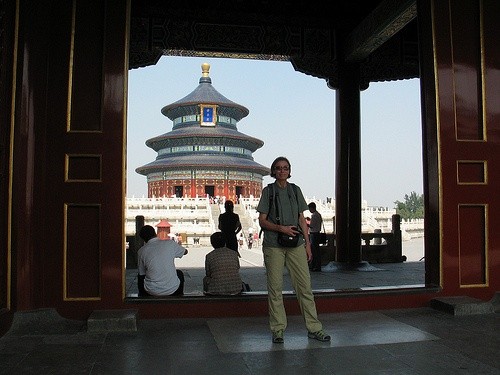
311;268;320;272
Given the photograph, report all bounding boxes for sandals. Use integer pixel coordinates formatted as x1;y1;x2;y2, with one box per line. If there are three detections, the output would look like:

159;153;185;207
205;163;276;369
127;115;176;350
272;330;284;342
308;330;331;340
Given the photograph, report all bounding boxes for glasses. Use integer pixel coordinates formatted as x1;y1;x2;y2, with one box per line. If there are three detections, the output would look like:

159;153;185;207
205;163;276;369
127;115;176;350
274;166;290;171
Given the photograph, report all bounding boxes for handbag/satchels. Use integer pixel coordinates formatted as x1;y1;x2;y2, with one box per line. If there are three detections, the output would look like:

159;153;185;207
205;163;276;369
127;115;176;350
277;229;299;247
242;282;250;292
318;233;327;244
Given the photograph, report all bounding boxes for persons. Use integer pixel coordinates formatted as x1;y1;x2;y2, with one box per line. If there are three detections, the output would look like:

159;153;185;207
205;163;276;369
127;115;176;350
217;200;242;252
304;202;322;272
238;232;258;249
256;157;331;344
137;225;188;296
210;197;221;204
203;232;252;295
